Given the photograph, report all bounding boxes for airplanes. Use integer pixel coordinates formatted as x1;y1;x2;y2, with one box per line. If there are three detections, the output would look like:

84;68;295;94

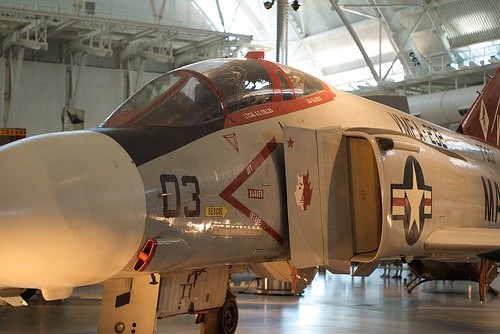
0;51;500;334
